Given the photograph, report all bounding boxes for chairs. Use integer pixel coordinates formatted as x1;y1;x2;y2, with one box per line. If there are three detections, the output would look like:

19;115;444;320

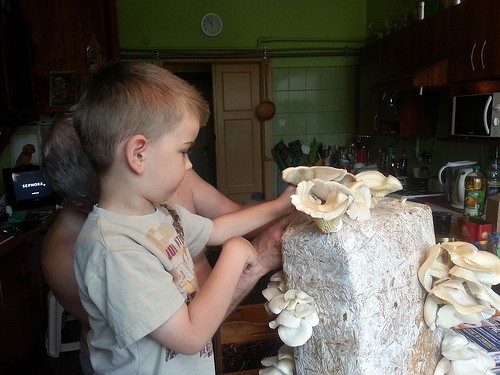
213;303;278;375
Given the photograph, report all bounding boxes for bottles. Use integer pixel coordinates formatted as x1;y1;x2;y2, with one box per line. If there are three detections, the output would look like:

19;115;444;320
324;143;407;177
485;164;500;195
463;165;487;220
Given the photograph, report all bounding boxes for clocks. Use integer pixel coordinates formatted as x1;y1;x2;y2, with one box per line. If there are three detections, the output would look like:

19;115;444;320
201;13;223;37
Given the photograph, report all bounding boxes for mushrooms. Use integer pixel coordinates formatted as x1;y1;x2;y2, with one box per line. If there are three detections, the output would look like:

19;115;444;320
282;165;403;233
258;271;319;375
418;242;500;375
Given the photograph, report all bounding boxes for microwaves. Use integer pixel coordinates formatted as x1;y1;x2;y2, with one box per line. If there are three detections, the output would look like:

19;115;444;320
451;92;500;138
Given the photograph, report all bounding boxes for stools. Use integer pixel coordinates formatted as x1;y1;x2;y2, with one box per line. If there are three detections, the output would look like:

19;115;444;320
46;290;81;358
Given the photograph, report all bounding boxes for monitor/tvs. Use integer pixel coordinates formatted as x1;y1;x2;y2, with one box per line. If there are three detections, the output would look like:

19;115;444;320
3;165;61;211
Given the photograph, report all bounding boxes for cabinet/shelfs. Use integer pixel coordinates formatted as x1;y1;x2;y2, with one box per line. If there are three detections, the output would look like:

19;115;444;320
0;205;59;370
357;0;500;137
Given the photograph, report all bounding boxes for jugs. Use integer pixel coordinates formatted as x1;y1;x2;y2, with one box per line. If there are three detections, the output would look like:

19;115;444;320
438;160;478;204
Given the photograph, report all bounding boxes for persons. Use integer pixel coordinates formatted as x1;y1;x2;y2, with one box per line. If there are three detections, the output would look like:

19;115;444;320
39;101;300;375
73;57;298;375
